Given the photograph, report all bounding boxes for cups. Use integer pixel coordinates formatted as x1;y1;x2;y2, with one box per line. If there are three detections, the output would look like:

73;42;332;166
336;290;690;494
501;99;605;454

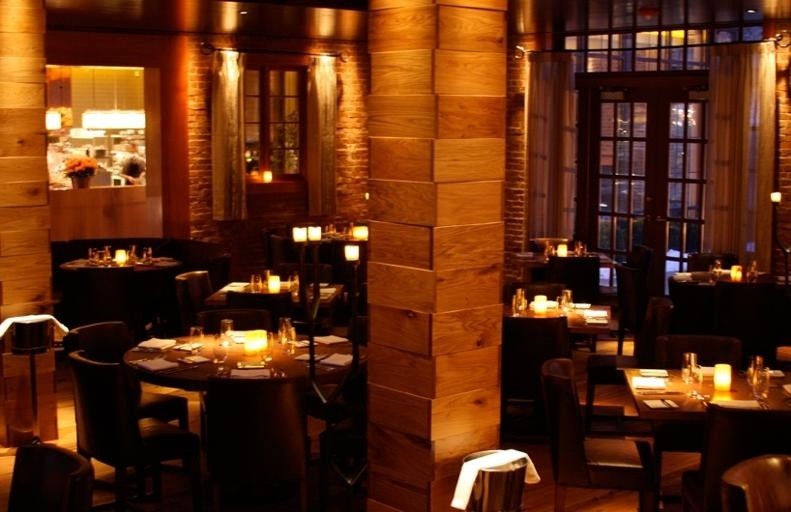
680;351;770;400
250;269;300;301
511;288;571;317
711;259;759;284
189;317;296;363
544;240;588;262
88;245;152;267
323;222;354;241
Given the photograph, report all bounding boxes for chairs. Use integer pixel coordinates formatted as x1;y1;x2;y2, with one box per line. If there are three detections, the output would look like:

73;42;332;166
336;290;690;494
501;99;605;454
497;233;791;510
0;215;370;512
